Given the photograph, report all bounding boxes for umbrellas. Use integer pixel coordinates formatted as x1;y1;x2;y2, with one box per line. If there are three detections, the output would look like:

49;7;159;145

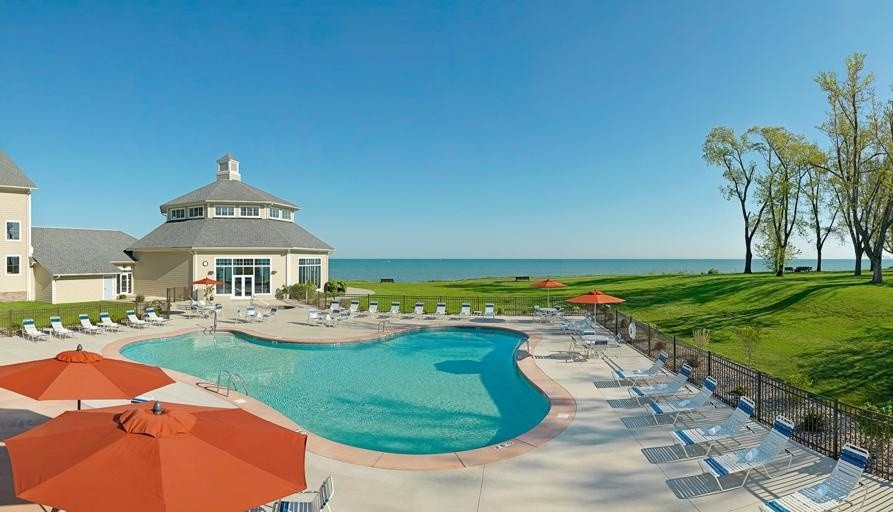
0;401;307;512
531;278;566;308
0;343;176;410
566;288;627;328
191;277;224;302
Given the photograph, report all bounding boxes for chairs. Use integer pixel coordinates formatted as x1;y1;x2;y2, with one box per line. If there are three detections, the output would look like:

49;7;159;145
627;363;693;407
233;296;499;330
270;472;339;512
644;376;718;427
611;351;669;389
673;396;756;459
758;442;870;510
703;415;796;493
18;293;222;344
529;299;624;363
